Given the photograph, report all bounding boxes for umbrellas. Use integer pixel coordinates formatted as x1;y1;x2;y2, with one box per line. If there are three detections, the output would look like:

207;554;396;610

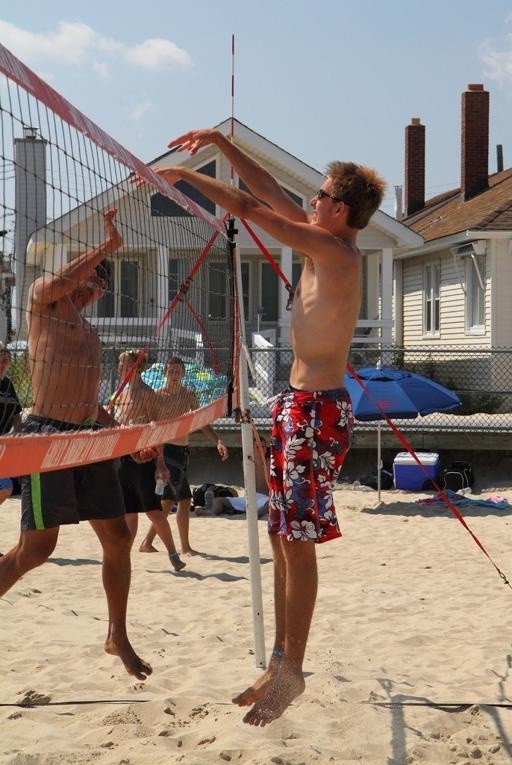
345;360;462;501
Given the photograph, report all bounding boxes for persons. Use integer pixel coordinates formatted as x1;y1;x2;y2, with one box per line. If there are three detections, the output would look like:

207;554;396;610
0;348;23;556
117;349;186;571
138;357;228;556
126;129;386;725
0;209;151;680
211;488;270;520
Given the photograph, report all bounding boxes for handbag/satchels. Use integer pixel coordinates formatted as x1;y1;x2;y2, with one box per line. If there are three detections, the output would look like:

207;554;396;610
442;461;475;492
193;484;237;507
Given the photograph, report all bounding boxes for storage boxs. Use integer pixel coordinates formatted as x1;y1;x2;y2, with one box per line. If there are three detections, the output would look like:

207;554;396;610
393;452;440;492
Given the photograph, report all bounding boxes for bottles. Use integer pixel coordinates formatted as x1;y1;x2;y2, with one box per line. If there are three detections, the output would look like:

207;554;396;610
205;488;215;511
154;471;166;496
458;487;471;496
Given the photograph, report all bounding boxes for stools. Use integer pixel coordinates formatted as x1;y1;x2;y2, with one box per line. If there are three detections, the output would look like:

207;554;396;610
440;462;475;489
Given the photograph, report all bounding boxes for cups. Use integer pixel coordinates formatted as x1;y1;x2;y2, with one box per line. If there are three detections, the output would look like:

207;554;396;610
195;506;202;516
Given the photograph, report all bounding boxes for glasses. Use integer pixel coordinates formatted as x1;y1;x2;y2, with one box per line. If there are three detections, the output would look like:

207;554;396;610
316;190;351;207
